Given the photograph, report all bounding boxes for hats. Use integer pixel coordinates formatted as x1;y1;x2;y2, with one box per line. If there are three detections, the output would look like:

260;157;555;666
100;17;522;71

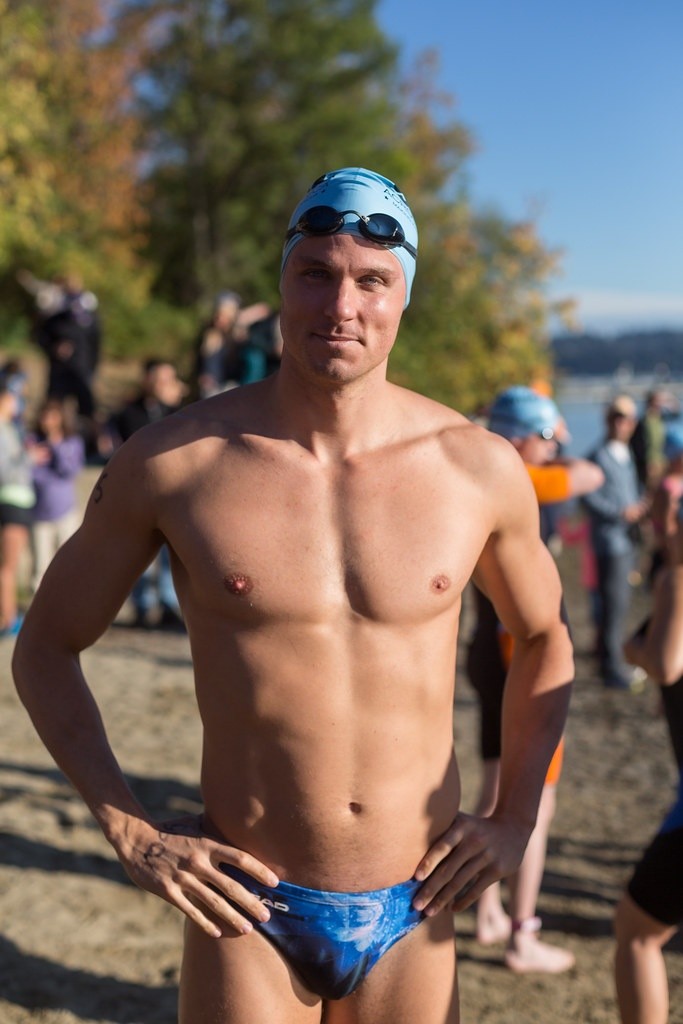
281;165;418;310
488;385;559;439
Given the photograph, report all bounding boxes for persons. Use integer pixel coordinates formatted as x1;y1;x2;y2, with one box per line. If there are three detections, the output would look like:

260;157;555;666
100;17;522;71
0;268;289;645
462;380;683;1024
10;167;576;1024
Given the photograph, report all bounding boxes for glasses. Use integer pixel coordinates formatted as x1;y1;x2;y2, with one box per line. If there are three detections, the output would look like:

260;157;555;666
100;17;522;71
287;205;418;260
